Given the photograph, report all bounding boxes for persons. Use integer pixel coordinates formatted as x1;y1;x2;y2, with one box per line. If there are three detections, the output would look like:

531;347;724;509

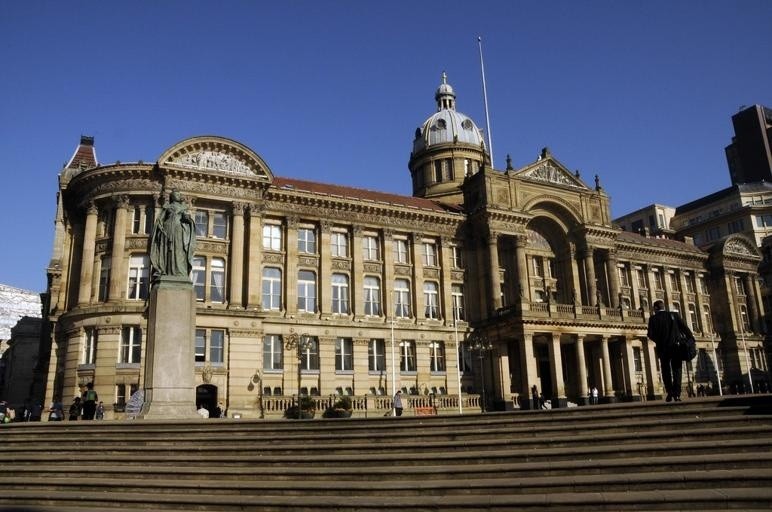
149;191;196;277
648;301;696;401
532;385;539;409
394;391;403;416
7;383;105;421
592;387;599;404
539;393;547;409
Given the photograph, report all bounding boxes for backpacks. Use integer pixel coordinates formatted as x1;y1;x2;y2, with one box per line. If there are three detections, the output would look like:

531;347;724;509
669;314;696;362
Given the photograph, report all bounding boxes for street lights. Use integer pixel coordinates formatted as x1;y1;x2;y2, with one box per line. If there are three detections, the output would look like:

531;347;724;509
466;334;494;413
284;332;314;420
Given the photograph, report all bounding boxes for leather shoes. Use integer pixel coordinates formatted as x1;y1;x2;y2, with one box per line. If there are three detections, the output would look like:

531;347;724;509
665;389;682;403
